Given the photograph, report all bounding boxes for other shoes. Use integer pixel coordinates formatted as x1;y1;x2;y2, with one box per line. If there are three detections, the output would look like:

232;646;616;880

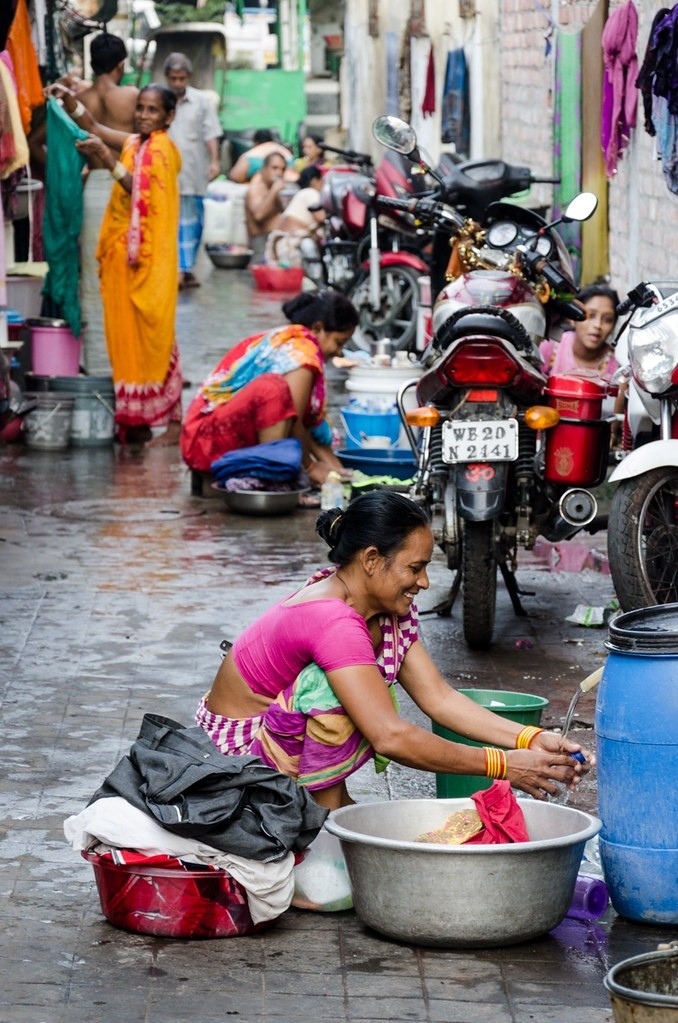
183;274;200;288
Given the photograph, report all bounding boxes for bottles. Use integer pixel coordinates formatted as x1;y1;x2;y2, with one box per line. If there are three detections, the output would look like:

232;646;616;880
349;399;366;410
567;874;610;921
385;399;400;415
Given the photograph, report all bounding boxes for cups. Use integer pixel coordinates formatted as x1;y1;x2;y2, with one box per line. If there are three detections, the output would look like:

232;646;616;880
372;341;392;368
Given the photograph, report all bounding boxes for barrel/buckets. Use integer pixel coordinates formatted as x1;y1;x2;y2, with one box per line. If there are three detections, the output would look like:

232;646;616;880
432;689;549;799
416;276;434;351
594;602;678;925
5;276;115;448
340;364;427;449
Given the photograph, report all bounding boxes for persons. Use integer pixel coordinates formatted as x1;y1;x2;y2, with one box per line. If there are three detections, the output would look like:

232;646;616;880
163;53;224;290
193;491;598;814
539;284;621;378
228;128;327;264
41;81;181;444
64;33;141;375
180;290;356;488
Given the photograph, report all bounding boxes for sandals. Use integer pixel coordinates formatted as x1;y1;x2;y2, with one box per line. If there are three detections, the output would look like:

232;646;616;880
297;493;321;509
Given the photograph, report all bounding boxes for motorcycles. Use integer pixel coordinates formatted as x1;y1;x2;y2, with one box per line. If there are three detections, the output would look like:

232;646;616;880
301;116;598;649
606;280;678;612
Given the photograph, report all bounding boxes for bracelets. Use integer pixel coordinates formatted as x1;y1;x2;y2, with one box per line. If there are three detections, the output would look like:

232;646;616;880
68;100;85;119
111;160;128;181
484;745;507;781
515;725;544;750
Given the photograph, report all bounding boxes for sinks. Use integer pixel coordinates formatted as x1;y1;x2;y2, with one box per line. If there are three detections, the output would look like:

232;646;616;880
10;178;42;222
5;266;45;327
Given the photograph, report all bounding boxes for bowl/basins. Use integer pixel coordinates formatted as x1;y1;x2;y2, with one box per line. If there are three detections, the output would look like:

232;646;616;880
334;447;418;482
81;849;260;939
248;263;305;290
12;178;44;221
209;248;254;266
601;947;678;1023
324;797;603;946
210;480;312;513
